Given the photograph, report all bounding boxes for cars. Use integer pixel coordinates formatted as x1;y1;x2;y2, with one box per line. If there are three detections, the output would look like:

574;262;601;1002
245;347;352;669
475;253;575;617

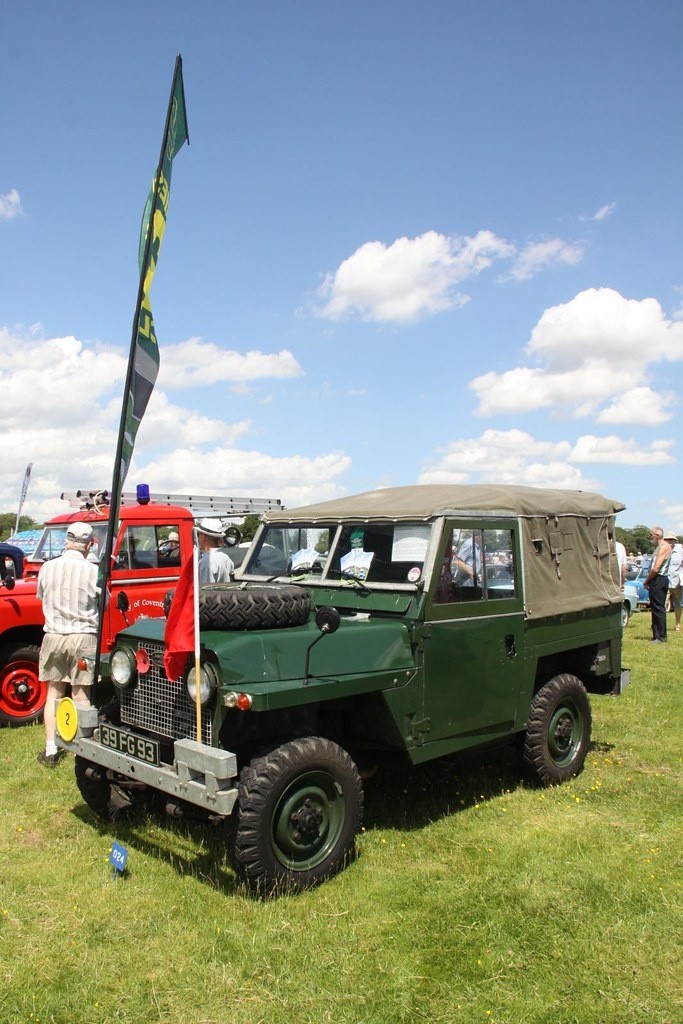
621;584;639;627
626;564;654;609
474;564;514;589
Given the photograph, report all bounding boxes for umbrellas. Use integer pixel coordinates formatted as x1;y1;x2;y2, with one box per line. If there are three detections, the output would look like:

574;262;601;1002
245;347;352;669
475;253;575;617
4;529;93;552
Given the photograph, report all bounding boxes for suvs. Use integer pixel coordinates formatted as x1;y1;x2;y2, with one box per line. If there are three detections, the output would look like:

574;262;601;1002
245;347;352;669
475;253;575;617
52;484;626;897
0;489;288;724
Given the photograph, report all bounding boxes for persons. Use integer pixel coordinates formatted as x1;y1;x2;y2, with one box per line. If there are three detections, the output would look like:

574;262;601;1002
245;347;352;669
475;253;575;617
496;555;506;566
663;532;683;632
194;518;235;585
457;529;485;587
629;552;643;562
616;541;626;593
644;527;671;644
37;522;111;766
158;532;178;550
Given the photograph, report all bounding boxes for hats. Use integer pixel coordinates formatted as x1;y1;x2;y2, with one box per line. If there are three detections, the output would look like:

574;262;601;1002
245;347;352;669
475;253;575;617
66;521;99;544
193;518;226;538
663;532;678;541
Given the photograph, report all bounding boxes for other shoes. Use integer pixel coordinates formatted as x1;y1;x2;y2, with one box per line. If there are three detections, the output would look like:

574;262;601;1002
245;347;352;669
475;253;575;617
675;624;680;632
43;747;67;766
651;639;662;644
